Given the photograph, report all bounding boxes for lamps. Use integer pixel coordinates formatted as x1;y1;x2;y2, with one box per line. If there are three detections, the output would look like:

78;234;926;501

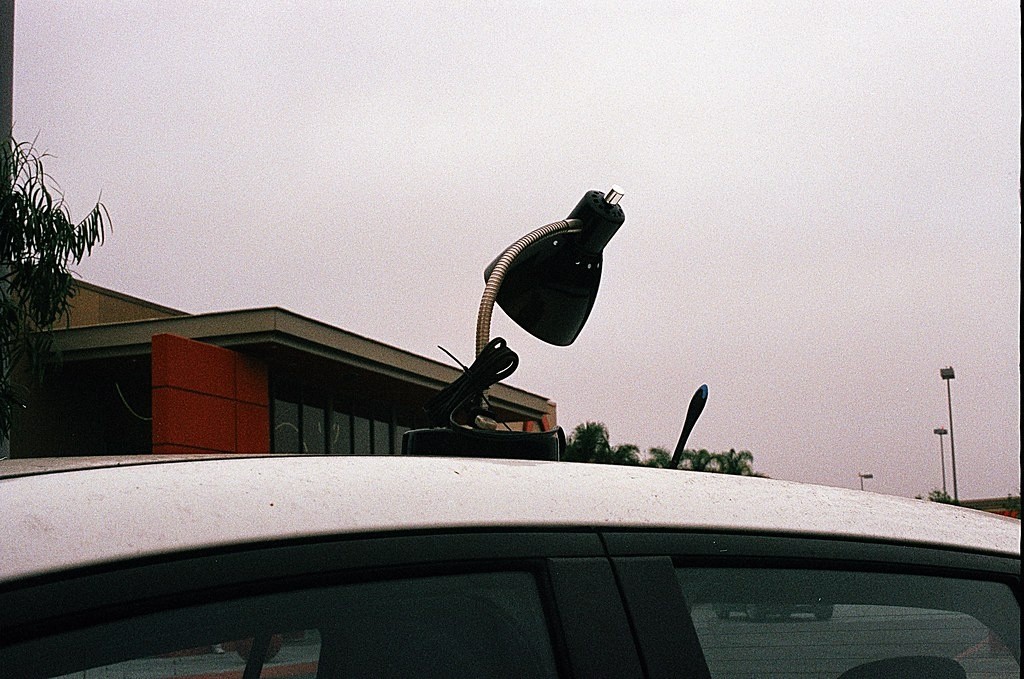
400;184;628;461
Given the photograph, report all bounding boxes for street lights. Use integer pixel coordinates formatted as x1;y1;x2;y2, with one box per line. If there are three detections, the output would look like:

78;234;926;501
935;428;948;503
939;365;959;501
860;472;872;490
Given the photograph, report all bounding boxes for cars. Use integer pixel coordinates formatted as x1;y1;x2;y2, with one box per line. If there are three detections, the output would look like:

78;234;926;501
0;451;1023;679
713;598;832;621
